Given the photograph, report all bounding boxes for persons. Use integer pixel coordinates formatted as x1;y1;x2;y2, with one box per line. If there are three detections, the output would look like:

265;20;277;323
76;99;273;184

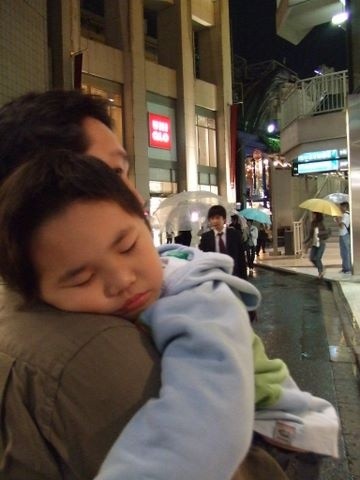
301;212;331;279
242;218;259;272
198;205;246;282
256;223;266;256
0;89;320;480
333;202;353;274
229;215;241;233
165;231;175;244
174;216;192;247
0;144;340;480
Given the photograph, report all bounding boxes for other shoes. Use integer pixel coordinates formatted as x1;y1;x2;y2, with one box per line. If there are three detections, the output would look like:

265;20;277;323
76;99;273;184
340;271;351;275
319;271;324;280
248;267;254;278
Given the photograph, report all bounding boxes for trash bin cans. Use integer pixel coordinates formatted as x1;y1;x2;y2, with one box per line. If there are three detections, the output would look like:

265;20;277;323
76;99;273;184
284;232;294;255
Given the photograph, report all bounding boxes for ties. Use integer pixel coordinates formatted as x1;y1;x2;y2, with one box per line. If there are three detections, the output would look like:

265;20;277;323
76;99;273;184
218;232;225;253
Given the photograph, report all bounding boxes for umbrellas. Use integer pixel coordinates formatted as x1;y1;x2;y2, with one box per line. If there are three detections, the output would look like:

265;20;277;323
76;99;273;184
149;190;233;232
298;198;343;217
323;192;349;203
238;208;272;224
